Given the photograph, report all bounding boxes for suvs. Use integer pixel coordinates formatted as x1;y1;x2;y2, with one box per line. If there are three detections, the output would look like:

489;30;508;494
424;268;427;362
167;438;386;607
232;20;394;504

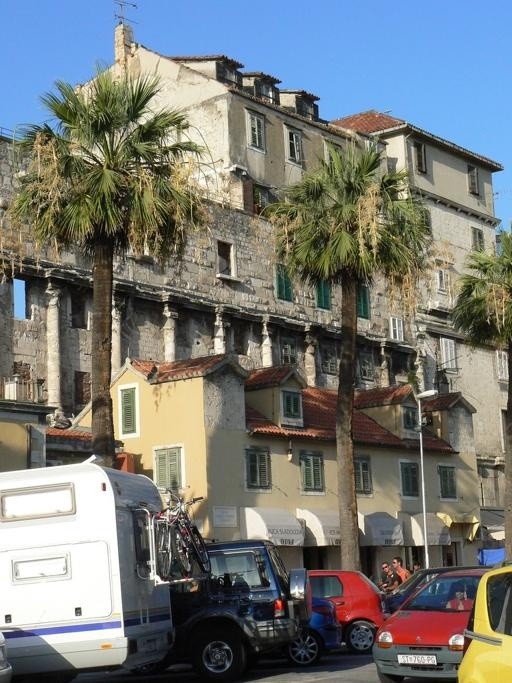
158;536;314;681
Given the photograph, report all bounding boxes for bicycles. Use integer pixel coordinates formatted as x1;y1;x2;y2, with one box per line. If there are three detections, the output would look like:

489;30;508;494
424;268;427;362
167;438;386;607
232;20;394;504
139;495;214;579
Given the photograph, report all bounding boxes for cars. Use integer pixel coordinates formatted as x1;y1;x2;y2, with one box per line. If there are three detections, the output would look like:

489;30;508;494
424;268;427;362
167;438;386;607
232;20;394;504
372;567;498;682
385;565;494;616
456;562;512;683
131;596;342;676
1;630;13;682
306;568;386;655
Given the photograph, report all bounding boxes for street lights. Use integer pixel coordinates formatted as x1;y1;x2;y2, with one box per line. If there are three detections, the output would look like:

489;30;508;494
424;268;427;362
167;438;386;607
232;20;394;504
415;389;438;594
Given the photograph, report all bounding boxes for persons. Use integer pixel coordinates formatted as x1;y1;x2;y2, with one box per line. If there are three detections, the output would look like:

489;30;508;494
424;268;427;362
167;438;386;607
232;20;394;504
413;562;421;572
376;561;402;591
392;556;411;583
445;582;474;611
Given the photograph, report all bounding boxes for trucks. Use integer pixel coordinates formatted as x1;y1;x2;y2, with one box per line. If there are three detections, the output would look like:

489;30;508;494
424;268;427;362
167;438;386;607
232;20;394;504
1;453;178;682
